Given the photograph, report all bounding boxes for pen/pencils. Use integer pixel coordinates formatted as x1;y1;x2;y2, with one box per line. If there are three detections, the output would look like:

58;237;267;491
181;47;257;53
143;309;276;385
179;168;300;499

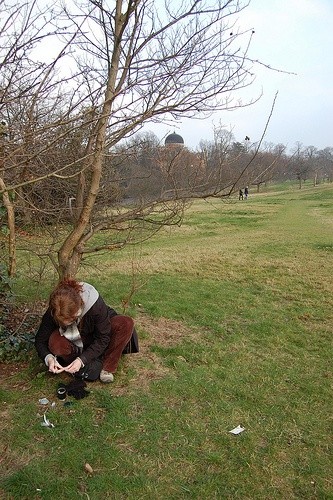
53;355;56;374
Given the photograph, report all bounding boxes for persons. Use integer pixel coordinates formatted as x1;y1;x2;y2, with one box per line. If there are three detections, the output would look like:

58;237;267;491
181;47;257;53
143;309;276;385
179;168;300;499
239;186;248;200
34;278;139;383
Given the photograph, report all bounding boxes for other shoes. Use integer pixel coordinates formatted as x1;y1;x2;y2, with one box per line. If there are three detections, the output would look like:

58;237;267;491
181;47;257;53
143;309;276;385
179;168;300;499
99;369;114;384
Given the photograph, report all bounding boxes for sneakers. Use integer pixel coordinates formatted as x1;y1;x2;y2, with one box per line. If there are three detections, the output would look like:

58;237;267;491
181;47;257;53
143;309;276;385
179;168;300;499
57;382;67;402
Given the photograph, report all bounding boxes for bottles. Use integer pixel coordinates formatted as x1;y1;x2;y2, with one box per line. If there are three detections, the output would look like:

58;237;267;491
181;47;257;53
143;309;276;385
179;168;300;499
57;387;67;401
